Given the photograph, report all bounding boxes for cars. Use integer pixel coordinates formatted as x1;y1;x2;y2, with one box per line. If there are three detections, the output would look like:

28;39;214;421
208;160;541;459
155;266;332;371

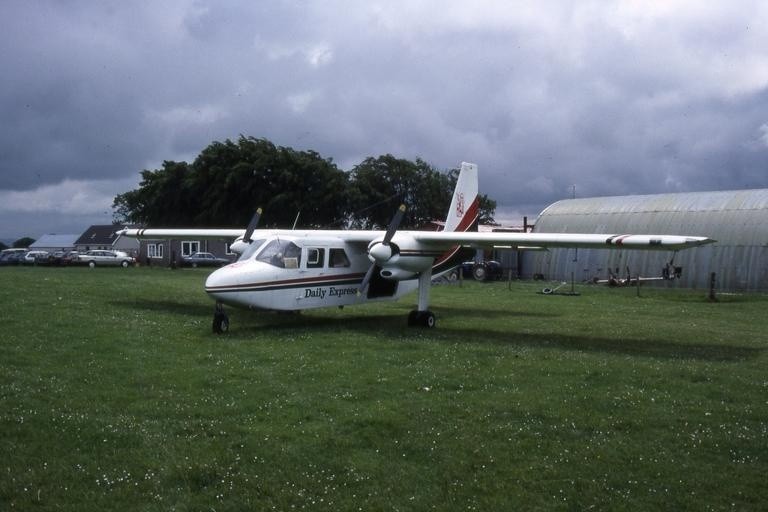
184;251;230;268
0;248;136;268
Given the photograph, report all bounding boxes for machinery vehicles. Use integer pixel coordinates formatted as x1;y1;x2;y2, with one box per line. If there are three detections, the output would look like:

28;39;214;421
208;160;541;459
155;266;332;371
450;260;503;281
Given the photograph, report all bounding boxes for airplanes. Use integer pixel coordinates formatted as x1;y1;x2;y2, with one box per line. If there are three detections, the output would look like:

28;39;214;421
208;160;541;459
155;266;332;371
115;162;718;335
